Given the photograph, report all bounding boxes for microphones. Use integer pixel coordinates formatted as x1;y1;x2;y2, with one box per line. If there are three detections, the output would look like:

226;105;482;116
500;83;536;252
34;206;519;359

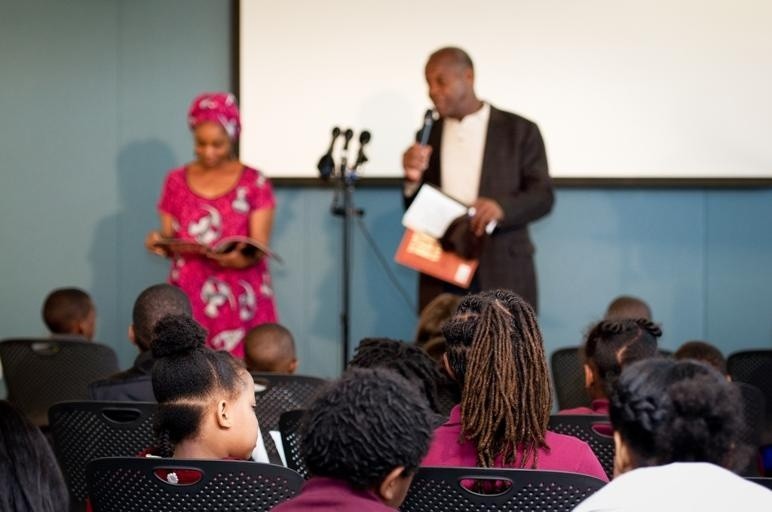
318;128;340;175
356;130;370;169
340;129;352;160
418;110;436;149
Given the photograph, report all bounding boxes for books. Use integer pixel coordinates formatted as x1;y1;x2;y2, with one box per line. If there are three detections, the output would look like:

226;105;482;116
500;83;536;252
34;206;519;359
153;235;282;264
394;227;482;289
401;182;498;242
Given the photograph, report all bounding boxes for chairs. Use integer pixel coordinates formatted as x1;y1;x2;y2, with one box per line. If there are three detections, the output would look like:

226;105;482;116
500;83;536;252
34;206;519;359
0;338;772;511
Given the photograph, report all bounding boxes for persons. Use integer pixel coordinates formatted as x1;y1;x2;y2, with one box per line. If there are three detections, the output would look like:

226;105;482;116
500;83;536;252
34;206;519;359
402;46;555;338
2;285;297;511
559;298;770;512
264;287;610;512
143;90;277;361
